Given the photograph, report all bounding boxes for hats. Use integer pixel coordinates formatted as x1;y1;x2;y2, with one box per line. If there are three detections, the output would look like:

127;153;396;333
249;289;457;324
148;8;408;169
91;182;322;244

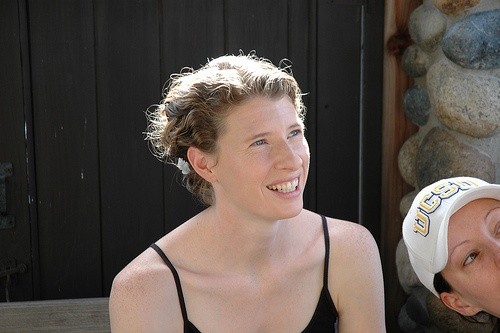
403;176;499;299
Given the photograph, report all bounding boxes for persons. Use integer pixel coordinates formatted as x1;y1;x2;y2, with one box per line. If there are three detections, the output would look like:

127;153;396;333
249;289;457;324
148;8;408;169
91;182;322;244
403;176;500;332
110;47;387;333
